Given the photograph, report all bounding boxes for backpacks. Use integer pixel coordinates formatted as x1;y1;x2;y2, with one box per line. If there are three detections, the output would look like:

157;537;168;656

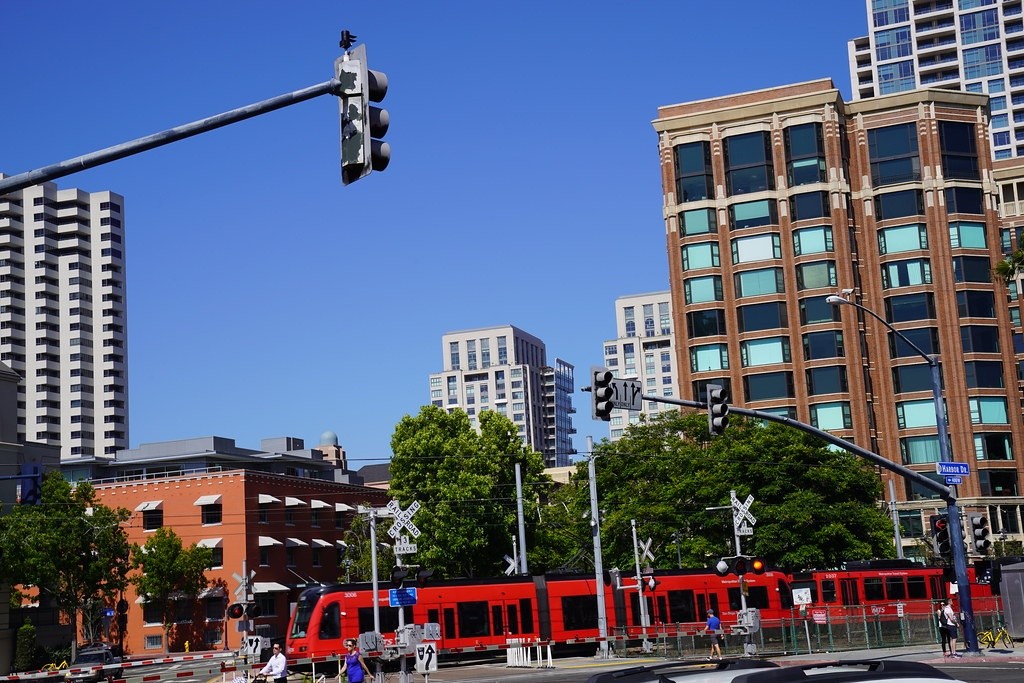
939;610;947;626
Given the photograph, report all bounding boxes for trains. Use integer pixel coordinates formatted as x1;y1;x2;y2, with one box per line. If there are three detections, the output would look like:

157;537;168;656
283;557;1004;677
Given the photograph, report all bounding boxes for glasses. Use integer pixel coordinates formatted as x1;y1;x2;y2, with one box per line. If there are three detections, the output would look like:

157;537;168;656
346;644;353;647
273;647;280;650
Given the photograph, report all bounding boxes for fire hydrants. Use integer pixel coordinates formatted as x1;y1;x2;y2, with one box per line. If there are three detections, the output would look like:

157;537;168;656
183;640;190;654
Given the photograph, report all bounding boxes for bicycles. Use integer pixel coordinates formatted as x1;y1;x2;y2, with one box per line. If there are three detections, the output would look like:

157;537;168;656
978;620;1015;650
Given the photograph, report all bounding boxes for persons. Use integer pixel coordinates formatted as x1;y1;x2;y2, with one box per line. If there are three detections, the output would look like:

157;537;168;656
944;598;962;658
256;643;287;683
704;609;724;660
937;600;952;657
335;639;374;683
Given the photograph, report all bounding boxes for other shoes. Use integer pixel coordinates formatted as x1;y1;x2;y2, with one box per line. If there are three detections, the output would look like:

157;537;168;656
943;653;948;656
950;653;962;658
706;657;713;660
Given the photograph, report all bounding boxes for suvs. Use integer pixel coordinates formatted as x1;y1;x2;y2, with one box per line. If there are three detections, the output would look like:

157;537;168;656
587;655;968;683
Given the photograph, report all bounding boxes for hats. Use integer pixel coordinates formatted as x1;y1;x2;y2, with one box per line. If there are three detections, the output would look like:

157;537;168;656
707;609;713;613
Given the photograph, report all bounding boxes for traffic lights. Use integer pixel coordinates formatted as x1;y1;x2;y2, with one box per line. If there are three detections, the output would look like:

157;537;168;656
415;566;437;588
750;557;765;574
968;512;991;555
389;565;410;589
246;605;261;618
929;514;952;556
227;603;243;618
960;515;969;555
591;366;614;422
731;556;749;576
706;383;729;436
332;41;390;185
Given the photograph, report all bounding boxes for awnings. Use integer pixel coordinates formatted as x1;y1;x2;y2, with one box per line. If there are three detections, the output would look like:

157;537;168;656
134;544;158;555
259;536;353;548
197;587;224;598
197;538;223;548
134;500;163;511
193;494;222;505
358;506;376;513
285;497;306;506
335;503;355;511
311;500;332;508
259;494;281;504
253;582;289;593
135;592;152;604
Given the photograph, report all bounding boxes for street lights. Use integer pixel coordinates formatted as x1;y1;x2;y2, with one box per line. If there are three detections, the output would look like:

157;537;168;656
825;295;980;652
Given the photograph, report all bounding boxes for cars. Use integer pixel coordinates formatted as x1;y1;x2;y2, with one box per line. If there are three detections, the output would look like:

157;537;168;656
65;648;122;683
